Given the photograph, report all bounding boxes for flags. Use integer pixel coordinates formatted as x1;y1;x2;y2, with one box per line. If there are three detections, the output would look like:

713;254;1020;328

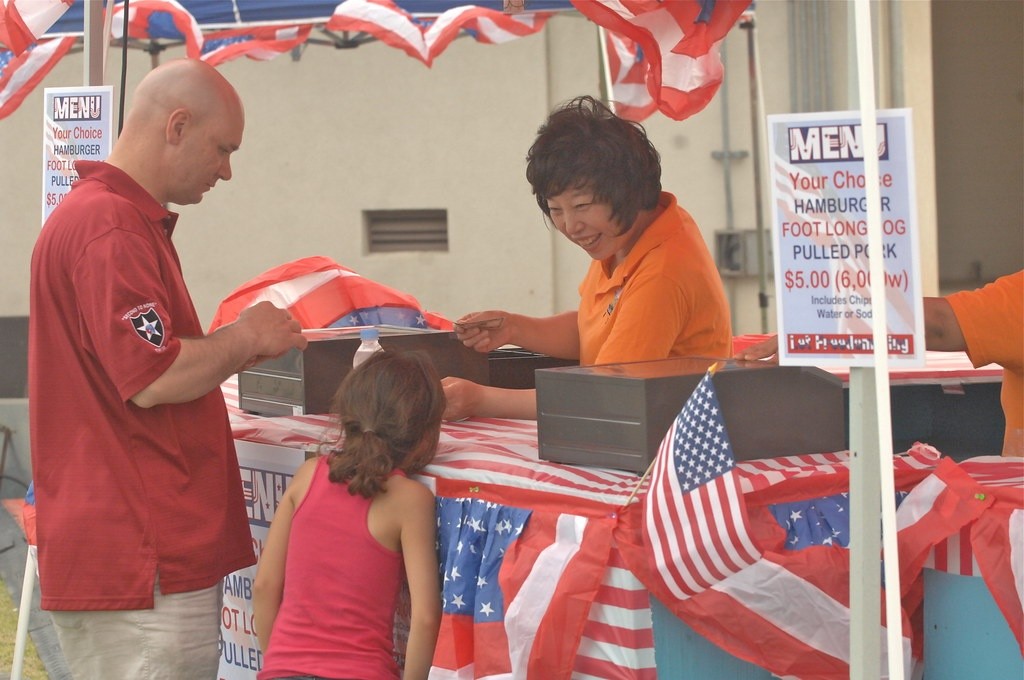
642;372;763;599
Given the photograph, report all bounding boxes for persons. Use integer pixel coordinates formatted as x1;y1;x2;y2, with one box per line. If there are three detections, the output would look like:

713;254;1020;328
253;350;447;680
733;268;1024;457
29;59;307;680
441;96;733;420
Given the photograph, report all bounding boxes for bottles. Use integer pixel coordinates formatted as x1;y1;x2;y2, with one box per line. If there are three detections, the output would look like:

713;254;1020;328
352;329;385;369
